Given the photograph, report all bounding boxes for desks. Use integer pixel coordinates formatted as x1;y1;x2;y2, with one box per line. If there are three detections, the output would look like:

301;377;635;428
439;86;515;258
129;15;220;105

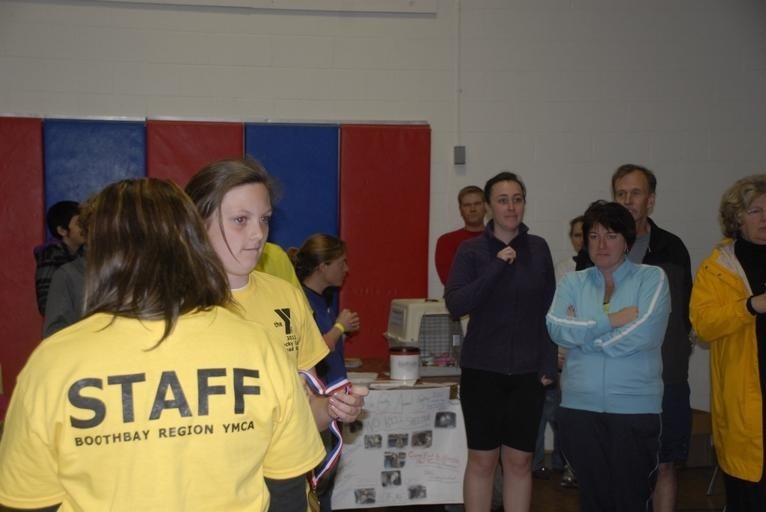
326;358;562;512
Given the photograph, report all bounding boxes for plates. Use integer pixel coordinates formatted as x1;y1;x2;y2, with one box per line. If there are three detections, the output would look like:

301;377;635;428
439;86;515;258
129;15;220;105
346;372;377;379
344;358;362;367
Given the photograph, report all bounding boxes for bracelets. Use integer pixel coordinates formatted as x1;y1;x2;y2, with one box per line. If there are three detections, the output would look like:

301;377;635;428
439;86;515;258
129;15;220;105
332;321;345;334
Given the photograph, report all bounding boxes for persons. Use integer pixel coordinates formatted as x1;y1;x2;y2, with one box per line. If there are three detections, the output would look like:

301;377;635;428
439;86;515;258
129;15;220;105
31;198;90;318
294;232;362;390
544;197;673;511
178;155;372;512
571;162;691;512
41;255;101;340
686;172;766;511
433;186;488;286
441;172;561;512
1;174;330;512
567;211;588;252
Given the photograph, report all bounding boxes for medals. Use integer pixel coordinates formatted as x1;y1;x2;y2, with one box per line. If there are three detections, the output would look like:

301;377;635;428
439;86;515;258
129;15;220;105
296;482;354;512
